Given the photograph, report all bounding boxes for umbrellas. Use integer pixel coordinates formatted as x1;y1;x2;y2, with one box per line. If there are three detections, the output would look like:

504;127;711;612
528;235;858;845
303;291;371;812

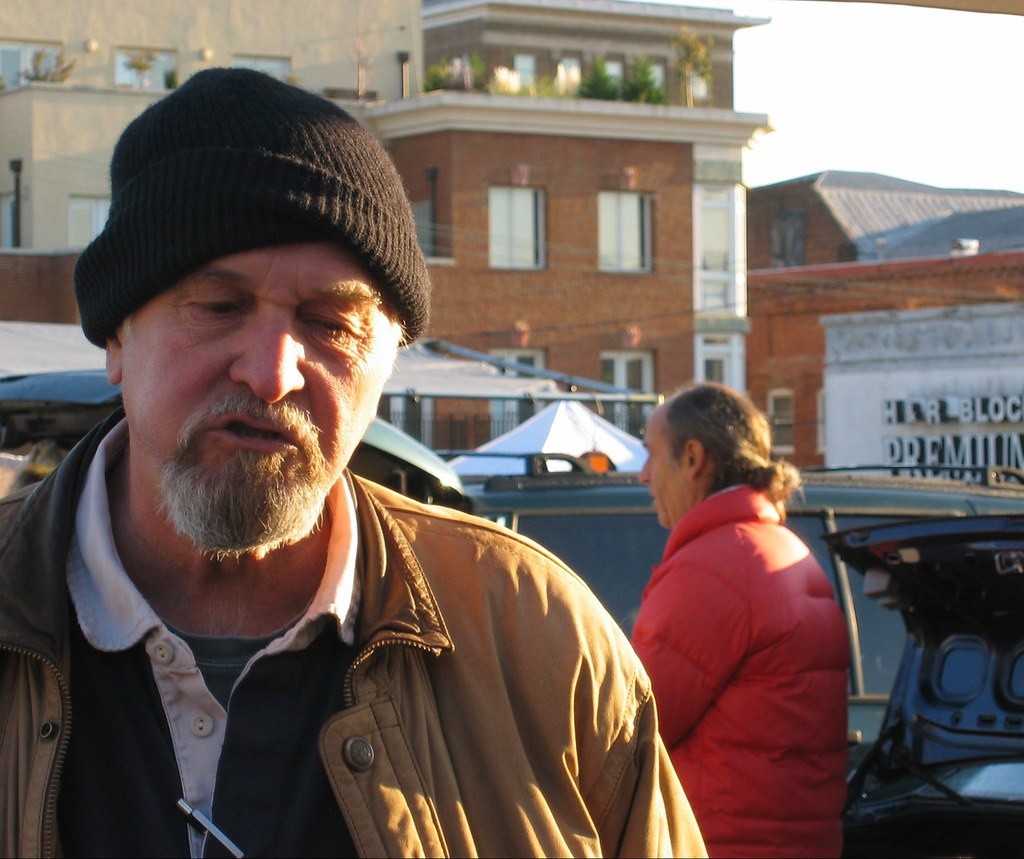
447;399;650;473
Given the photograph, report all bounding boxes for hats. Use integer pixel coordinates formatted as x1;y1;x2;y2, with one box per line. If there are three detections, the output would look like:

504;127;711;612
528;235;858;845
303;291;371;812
74;67;427;347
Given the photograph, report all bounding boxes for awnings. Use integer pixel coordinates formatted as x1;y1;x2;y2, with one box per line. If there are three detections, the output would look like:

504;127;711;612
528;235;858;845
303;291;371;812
0;370;124;406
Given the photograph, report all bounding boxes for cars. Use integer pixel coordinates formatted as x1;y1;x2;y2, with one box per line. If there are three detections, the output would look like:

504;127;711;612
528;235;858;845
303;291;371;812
819;515;1024;859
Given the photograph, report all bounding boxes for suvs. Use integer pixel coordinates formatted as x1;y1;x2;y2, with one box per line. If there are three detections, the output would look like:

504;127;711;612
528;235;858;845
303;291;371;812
446;450;1024;790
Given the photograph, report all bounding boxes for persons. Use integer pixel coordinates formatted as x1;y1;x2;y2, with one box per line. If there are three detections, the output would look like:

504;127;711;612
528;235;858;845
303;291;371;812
0;64;709;859
635;383;857;859
